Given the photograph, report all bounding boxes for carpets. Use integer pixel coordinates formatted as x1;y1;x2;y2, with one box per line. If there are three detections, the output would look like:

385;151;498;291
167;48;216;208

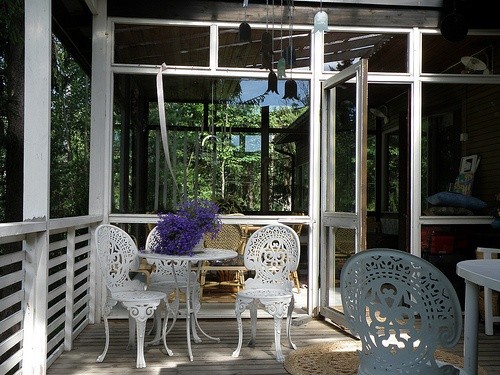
282;337;489;375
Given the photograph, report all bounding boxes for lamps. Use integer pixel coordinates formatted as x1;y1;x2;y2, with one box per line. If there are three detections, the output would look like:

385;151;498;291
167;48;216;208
371;102;392;125
460;47;493;78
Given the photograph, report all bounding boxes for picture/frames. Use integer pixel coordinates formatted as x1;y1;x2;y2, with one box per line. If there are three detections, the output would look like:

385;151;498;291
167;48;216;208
460;154;477;173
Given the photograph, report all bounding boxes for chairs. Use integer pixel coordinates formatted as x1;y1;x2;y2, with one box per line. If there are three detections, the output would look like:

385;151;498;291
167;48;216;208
228;223;301;362
244;210;307;294
339;245;461;375
141;222;203;343
200;224;245;301
477;244;500;335
92;222;177;369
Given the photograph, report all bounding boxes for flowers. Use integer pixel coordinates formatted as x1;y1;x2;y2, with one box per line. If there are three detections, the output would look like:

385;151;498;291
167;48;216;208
148;196;225;255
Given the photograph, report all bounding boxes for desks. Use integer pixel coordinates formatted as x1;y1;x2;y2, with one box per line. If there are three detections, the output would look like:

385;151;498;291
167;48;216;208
134;246;238;362
454;255;500;375
